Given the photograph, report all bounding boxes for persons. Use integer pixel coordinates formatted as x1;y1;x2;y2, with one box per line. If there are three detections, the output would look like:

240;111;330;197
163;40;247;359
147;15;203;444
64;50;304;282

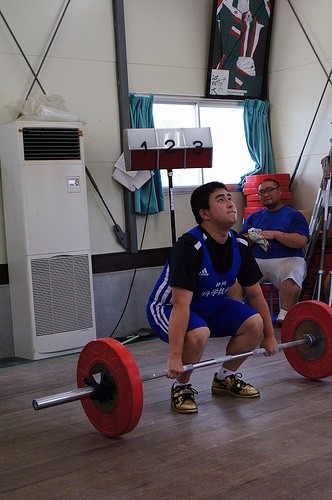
147;182;279;412
238;179;310;325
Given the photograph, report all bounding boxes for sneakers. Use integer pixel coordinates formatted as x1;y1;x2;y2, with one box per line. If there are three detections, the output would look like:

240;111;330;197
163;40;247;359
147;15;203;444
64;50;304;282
169;382;197;413
212;371;259;399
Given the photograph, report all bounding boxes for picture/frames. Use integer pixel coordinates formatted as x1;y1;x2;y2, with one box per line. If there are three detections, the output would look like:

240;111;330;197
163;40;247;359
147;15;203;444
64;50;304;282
205;0;276;101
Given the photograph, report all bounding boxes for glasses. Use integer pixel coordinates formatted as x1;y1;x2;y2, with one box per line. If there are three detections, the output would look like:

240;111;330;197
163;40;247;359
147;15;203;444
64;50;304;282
257;185;280;195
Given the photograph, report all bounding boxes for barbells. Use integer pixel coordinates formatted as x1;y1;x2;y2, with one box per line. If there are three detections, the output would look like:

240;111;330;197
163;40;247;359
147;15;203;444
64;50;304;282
30;299;332;441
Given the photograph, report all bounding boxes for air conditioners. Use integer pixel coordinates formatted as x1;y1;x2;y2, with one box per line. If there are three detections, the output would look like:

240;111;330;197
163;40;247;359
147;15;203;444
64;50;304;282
0;121;97;361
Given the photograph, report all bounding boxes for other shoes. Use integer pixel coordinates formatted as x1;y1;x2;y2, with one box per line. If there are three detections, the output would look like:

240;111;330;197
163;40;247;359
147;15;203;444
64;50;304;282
277;309;288;327
